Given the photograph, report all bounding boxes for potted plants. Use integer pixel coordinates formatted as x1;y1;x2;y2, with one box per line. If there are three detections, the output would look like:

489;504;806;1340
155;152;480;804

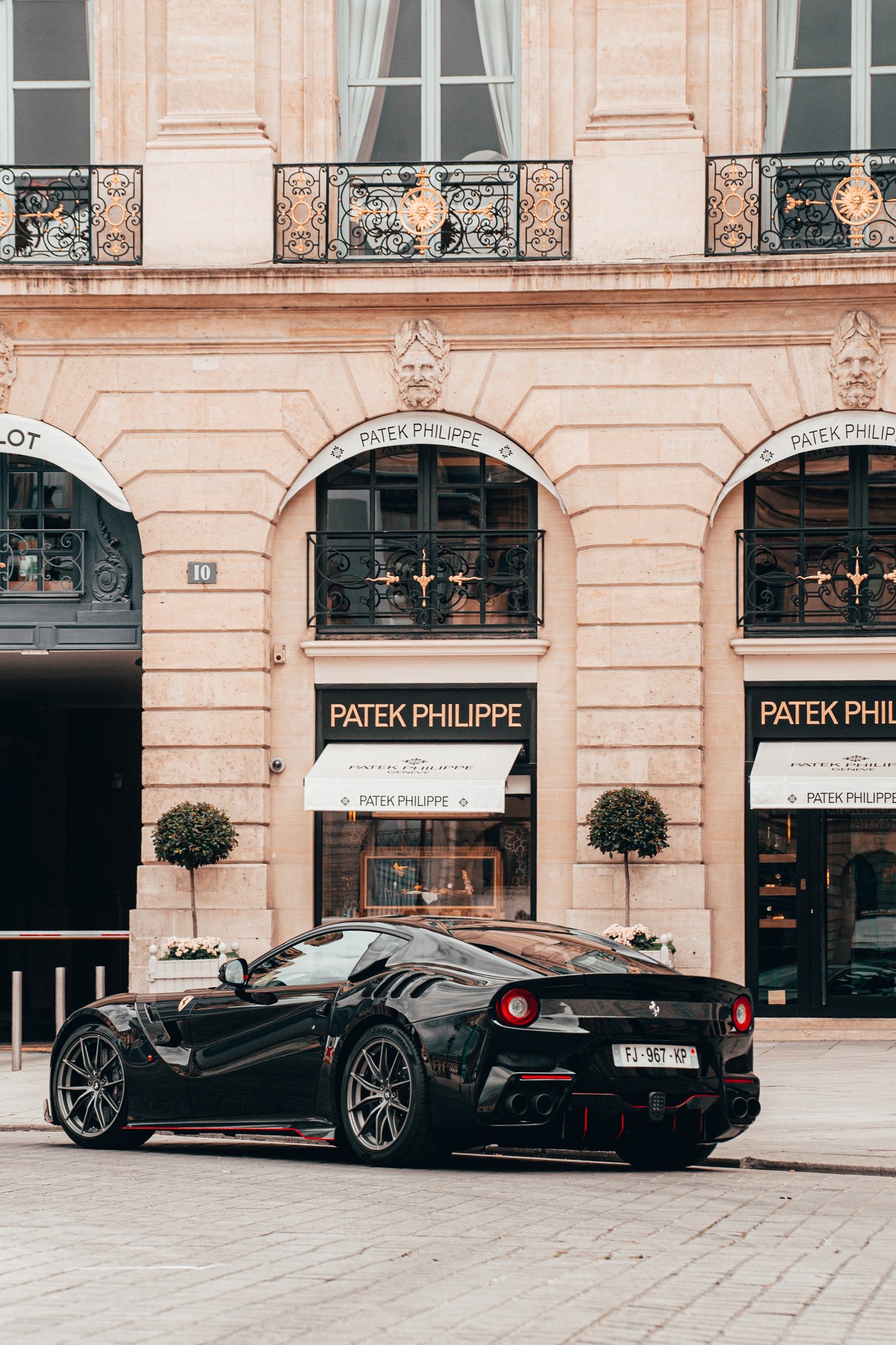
150;802;238;993
583;787;676;971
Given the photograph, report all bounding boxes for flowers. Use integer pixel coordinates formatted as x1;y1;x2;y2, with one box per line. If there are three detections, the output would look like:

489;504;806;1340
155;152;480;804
164;938;222;958
602;923;659;953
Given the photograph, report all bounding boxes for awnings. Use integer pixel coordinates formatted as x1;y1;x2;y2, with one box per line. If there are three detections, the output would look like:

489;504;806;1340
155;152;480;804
750;742;896;812
303;742;520;813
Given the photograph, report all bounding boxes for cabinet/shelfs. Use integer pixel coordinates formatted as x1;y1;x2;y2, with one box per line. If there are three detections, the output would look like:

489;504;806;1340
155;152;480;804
758;853;799;929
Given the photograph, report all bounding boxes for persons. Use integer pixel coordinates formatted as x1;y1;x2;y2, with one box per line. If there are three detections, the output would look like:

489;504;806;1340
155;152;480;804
827;311;888;409
386;320;454;411
0;324;17;412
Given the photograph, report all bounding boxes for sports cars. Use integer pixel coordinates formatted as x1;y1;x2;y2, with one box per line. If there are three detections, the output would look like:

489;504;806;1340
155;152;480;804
754;961;896;1019
48;915;762;1171
